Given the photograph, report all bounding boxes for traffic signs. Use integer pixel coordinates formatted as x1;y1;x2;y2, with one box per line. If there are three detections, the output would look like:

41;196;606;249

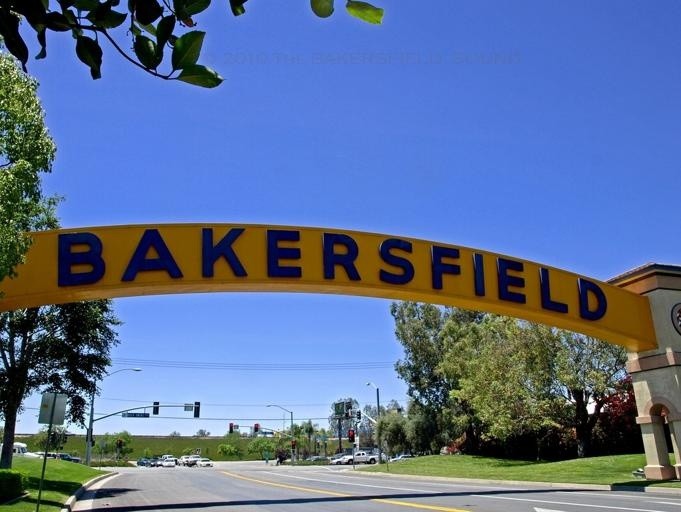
333;402;343;415
121;412;148;417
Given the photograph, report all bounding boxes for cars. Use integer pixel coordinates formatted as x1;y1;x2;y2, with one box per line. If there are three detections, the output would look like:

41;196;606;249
307;448;414;464
11;442;81;463
137;454;214;467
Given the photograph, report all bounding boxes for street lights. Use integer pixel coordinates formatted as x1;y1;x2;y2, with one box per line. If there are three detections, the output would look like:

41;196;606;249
366;380;383;463
86;367;142;466
267;404;296;465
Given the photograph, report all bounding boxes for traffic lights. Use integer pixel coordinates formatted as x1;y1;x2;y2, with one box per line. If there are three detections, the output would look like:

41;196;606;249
344;412;348;418
229;423;232;434
355;412;360;419
345;401;351;409
291;440;296;449
254;423;257;432
348;429;356;443
